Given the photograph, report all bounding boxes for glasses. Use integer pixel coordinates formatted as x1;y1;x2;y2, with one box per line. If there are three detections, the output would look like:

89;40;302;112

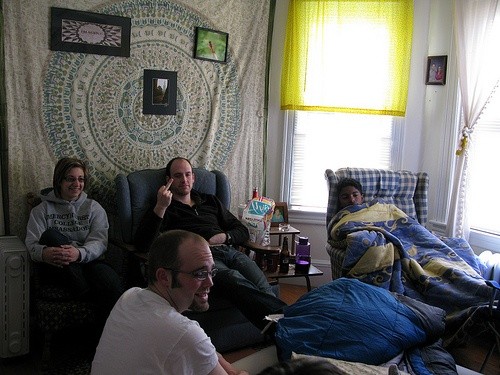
64;176;86;184
162;264;218;280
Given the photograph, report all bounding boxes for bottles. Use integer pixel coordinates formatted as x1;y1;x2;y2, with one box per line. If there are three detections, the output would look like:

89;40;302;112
280;236;289;273
295;237;311;269
252;186;258;199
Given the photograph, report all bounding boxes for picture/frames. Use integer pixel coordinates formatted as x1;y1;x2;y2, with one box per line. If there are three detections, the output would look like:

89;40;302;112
194;26;229;64
143;69;177;115
51;7;131;57
271;202;288;227
425;55;448;85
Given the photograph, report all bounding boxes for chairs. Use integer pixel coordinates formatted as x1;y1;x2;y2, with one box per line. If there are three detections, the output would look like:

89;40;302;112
24;188;122;373
325;167;430;281
115;167;277;354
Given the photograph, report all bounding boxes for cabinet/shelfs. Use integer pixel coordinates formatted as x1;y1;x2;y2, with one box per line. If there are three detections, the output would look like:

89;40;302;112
263;226;323;292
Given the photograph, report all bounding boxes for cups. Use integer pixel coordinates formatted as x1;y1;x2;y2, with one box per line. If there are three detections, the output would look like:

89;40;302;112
266;252;279;271
237;203;246;220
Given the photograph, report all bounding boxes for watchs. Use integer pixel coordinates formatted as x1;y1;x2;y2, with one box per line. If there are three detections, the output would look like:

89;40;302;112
226;232;232;244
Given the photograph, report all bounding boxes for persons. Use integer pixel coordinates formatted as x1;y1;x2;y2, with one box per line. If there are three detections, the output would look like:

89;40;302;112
133;156;287;338
24;157;109;368
332;178;494;319
91;229;248;375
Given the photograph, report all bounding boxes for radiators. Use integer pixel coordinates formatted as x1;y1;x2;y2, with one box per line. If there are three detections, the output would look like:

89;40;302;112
0;235;29;359
